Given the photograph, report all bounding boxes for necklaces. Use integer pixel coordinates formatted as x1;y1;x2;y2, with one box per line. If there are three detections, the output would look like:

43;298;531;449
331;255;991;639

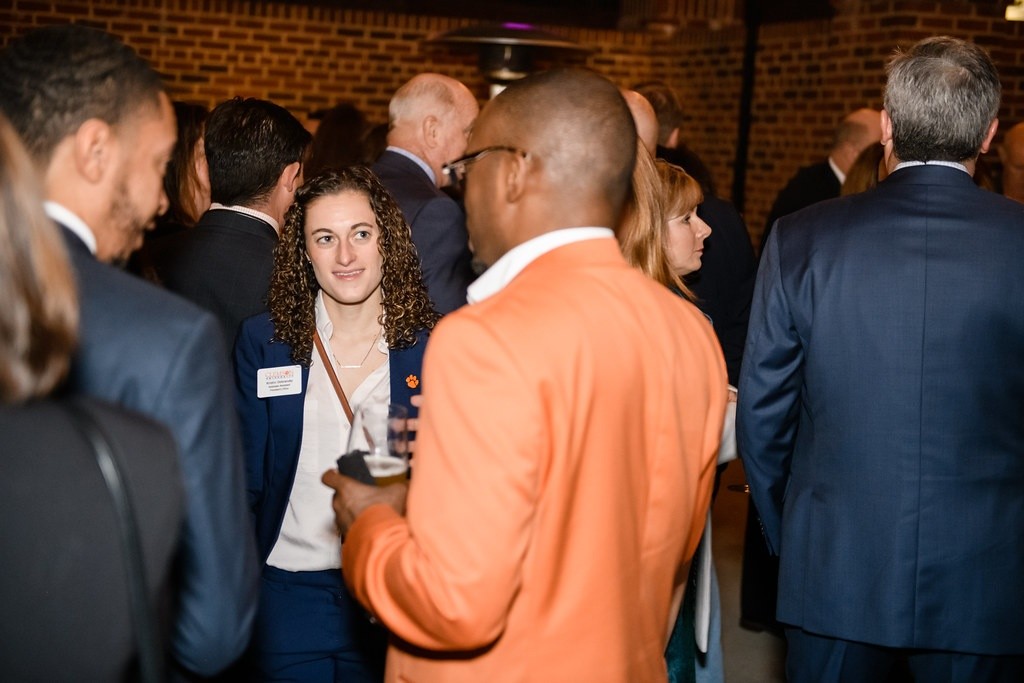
330;321;385;371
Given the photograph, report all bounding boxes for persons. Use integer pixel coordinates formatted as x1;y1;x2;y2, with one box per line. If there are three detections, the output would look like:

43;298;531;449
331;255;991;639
321;65;727;683
310;100;370;181
606;80;757;683
166;97;314;366
756;108;887;281
1;118;199;683
361;71;481;322
0;25;265;683
975;91;1024;204
126;101;212;303
230;166;444;683
735;39;1024;683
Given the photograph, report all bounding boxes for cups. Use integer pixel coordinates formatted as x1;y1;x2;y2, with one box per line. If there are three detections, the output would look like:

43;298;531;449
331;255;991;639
344;403;408;492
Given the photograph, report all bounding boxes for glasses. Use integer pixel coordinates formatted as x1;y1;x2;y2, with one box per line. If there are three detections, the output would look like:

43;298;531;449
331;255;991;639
442;145;527;194
